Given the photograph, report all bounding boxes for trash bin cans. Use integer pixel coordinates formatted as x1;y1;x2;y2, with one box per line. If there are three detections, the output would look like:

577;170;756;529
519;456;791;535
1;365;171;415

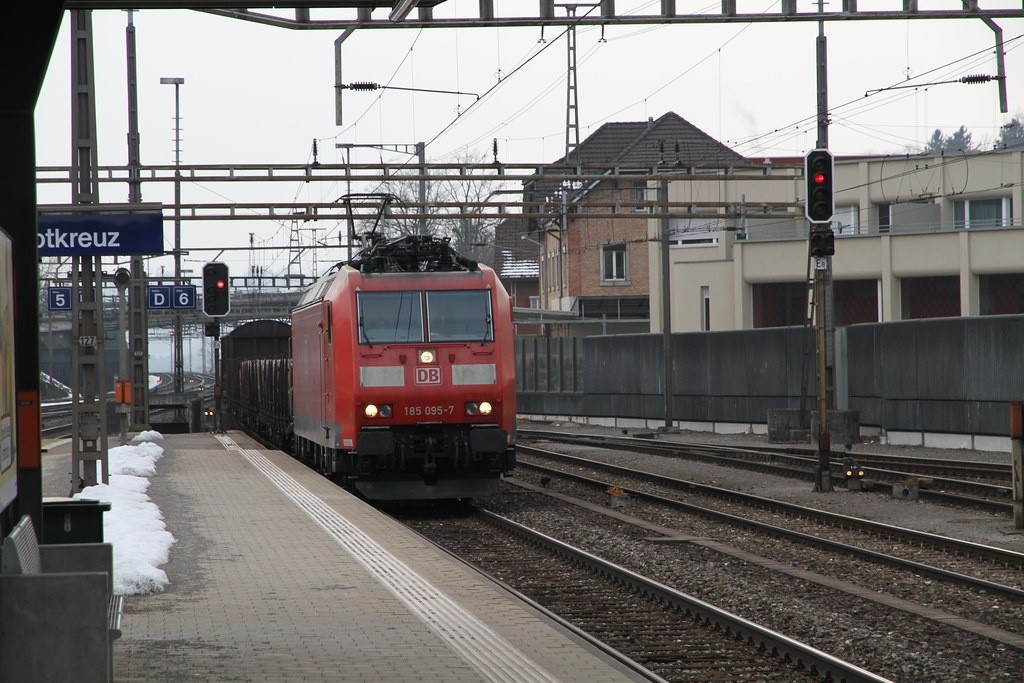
42;496;112;544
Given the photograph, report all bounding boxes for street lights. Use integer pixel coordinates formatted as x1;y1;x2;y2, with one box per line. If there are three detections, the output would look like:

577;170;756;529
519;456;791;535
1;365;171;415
160;76;186;424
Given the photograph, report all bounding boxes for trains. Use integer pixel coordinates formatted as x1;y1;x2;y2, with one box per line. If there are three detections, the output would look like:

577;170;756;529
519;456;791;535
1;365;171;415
217;190;519;505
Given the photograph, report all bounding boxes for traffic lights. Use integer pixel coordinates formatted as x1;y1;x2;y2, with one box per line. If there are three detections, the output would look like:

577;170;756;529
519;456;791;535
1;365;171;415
202;262;232;318
805;149;835;224
809;229;836;258
